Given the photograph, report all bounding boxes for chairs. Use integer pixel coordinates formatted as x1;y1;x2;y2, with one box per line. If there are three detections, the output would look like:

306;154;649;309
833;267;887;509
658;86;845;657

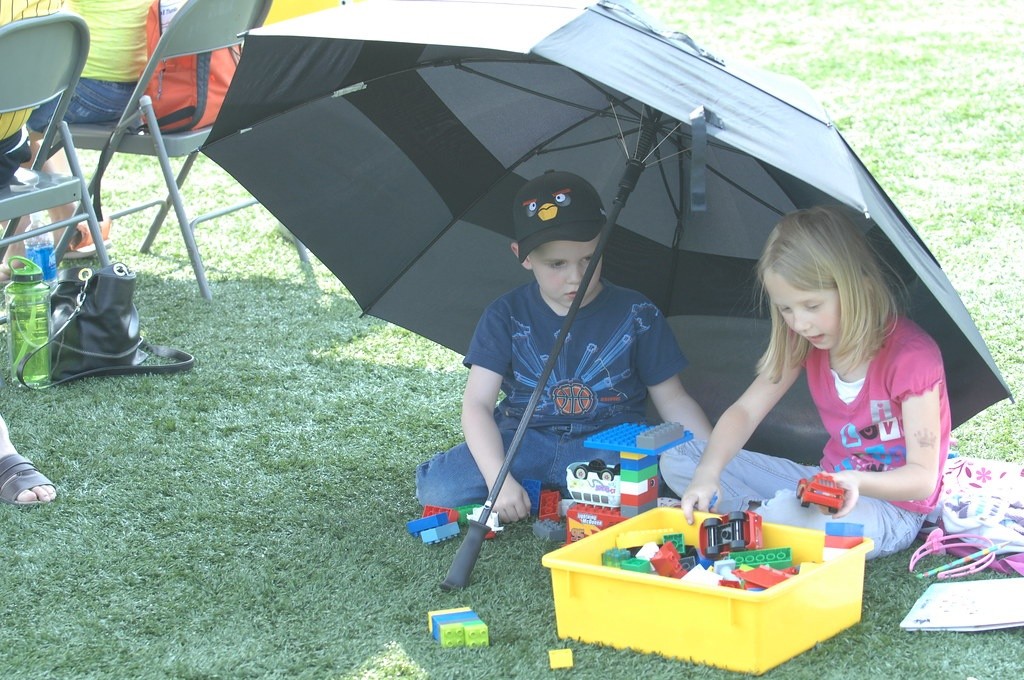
0;12;111;326
44;0;318;299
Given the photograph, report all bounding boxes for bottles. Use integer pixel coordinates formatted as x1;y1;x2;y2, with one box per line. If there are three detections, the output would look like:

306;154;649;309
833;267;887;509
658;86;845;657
5;254;50;387
24;213;59;294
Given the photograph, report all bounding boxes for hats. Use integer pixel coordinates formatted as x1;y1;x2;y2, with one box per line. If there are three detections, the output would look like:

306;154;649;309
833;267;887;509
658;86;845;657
510;168;608;264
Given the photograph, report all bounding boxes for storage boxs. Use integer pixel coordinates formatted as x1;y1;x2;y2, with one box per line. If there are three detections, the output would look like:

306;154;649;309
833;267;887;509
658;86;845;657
540;506;874;676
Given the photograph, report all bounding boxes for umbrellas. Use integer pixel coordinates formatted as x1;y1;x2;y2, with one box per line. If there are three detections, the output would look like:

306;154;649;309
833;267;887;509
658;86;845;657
193;0;1014;590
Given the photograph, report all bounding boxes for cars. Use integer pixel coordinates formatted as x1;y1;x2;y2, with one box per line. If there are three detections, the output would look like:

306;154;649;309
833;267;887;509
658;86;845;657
796;475;845;515
698;509;764;558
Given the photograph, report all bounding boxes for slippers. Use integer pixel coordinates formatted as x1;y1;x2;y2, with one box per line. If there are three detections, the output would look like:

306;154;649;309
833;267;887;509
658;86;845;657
1;453;58;509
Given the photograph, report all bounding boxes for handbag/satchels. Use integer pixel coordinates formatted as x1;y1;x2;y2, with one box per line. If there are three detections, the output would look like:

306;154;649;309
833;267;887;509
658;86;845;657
146;0;250;134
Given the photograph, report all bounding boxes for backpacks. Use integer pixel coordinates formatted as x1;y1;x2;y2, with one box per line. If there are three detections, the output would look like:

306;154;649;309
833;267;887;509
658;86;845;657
16;262;194;392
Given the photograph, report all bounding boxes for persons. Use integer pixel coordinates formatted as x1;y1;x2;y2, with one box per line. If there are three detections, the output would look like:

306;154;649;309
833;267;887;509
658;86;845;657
660;207;951;560
0;0;242;506
416;171;712;509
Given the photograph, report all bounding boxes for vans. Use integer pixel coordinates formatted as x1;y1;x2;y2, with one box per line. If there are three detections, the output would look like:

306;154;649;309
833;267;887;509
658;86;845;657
566;458;622;508
